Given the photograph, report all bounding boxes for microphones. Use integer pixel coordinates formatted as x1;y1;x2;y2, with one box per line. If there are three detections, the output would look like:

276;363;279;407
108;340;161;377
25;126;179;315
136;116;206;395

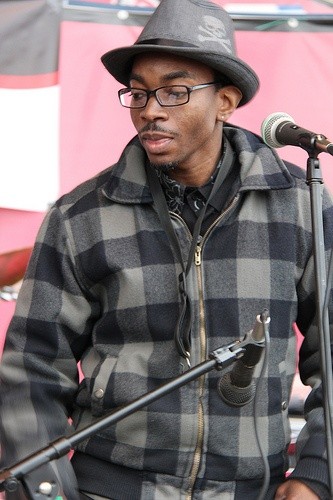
261;112;332;155
218;316;270;407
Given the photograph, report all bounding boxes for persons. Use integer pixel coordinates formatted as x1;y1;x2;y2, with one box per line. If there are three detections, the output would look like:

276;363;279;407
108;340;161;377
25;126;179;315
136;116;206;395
0;0;331;499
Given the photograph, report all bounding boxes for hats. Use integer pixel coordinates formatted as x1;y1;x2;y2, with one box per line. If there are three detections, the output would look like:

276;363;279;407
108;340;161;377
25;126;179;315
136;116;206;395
99;0;260;108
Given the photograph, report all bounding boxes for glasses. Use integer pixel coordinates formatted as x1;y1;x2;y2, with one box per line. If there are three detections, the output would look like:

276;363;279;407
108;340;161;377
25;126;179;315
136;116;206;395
117;81;218;109
